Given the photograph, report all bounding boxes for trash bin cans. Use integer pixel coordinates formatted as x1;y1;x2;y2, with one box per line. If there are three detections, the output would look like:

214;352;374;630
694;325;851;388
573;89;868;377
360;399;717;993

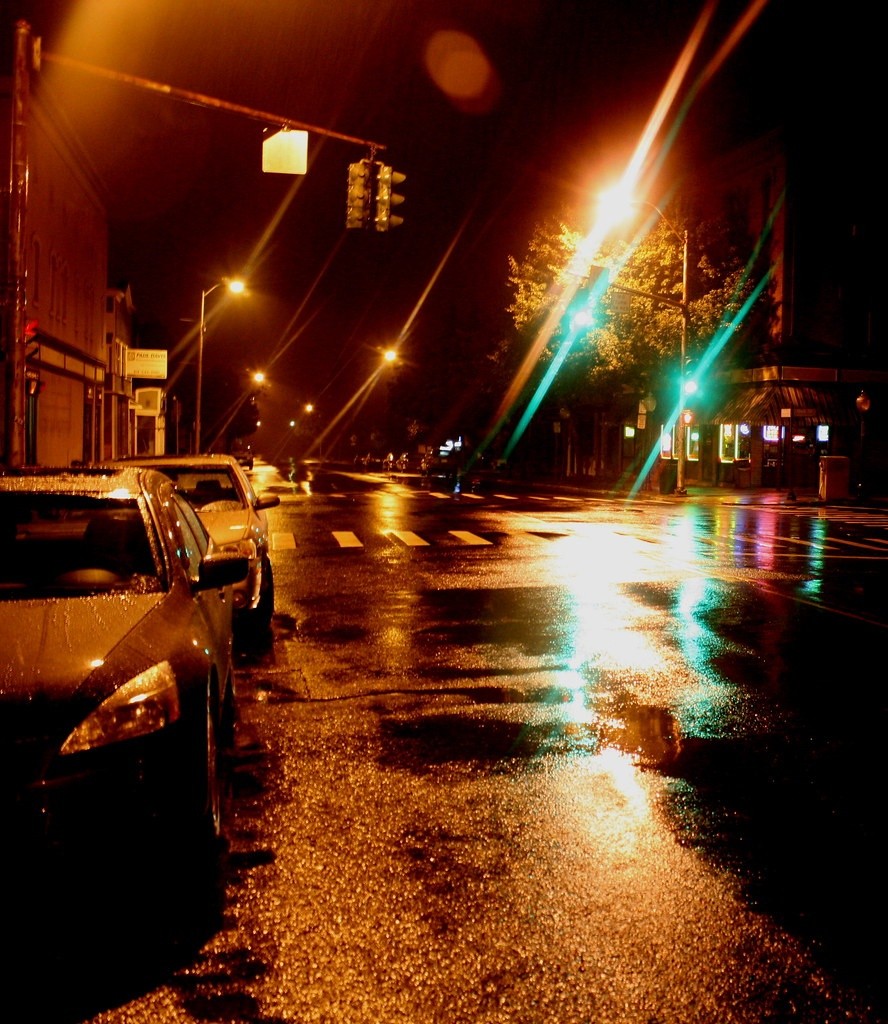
818;455;849;502
734;466;751;488
659;459;678;495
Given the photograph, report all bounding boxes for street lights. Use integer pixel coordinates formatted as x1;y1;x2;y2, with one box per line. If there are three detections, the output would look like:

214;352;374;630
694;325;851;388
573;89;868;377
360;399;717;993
194;274;246;454
602;193;687;495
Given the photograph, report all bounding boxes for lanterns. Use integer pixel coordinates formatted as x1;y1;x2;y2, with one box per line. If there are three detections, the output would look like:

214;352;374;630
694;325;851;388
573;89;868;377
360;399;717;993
856;394;870;412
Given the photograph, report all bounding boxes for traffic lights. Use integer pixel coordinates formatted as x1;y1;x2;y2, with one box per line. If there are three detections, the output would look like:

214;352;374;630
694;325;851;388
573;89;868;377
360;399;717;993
375;165;406;233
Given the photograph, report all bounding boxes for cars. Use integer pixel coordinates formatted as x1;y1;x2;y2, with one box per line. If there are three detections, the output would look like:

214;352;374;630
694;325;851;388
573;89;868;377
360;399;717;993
1;463;250;843
92;451;281;635
